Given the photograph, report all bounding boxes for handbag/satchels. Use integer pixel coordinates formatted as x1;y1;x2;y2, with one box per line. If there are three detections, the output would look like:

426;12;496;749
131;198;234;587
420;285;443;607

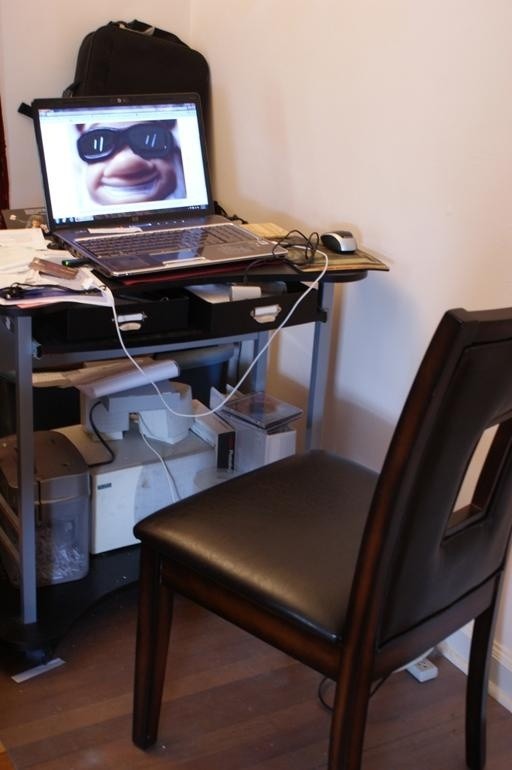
60;17;211;144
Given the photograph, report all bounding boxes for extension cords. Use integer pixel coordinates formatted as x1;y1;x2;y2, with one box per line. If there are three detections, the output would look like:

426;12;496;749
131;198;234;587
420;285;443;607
406;658;438;682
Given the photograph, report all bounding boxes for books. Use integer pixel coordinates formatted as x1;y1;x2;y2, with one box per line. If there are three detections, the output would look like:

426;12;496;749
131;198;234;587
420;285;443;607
241;223;292;239
223;391;303;434
0;206;114;307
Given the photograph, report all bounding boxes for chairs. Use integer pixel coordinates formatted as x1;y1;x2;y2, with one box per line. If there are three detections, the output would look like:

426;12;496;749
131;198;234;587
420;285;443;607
131;307;510;768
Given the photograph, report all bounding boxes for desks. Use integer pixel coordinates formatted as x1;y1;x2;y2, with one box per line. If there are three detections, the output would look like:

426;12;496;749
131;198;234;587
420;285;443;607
0;209;366;670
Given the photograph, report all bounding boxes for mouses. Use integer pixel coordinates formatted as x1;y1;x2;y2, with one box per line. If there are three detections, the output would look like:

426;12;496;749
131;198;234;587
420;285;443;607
320;231;356;253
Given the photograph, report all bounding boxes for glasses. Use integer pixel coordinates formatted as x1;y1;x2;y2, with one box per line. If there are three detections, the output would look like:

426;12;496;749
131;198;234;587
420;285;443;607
76;123;173;163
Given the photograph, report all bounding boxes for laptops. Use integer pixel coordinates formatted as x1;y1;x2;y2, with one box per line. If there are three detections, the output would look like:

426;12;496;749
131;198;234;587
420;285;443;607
31;93;288;280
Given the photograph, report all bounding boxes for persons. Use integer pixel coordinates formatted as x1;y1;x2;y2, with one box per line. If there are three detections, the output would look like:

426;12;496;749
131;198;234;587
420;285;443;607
75;119;187;206
